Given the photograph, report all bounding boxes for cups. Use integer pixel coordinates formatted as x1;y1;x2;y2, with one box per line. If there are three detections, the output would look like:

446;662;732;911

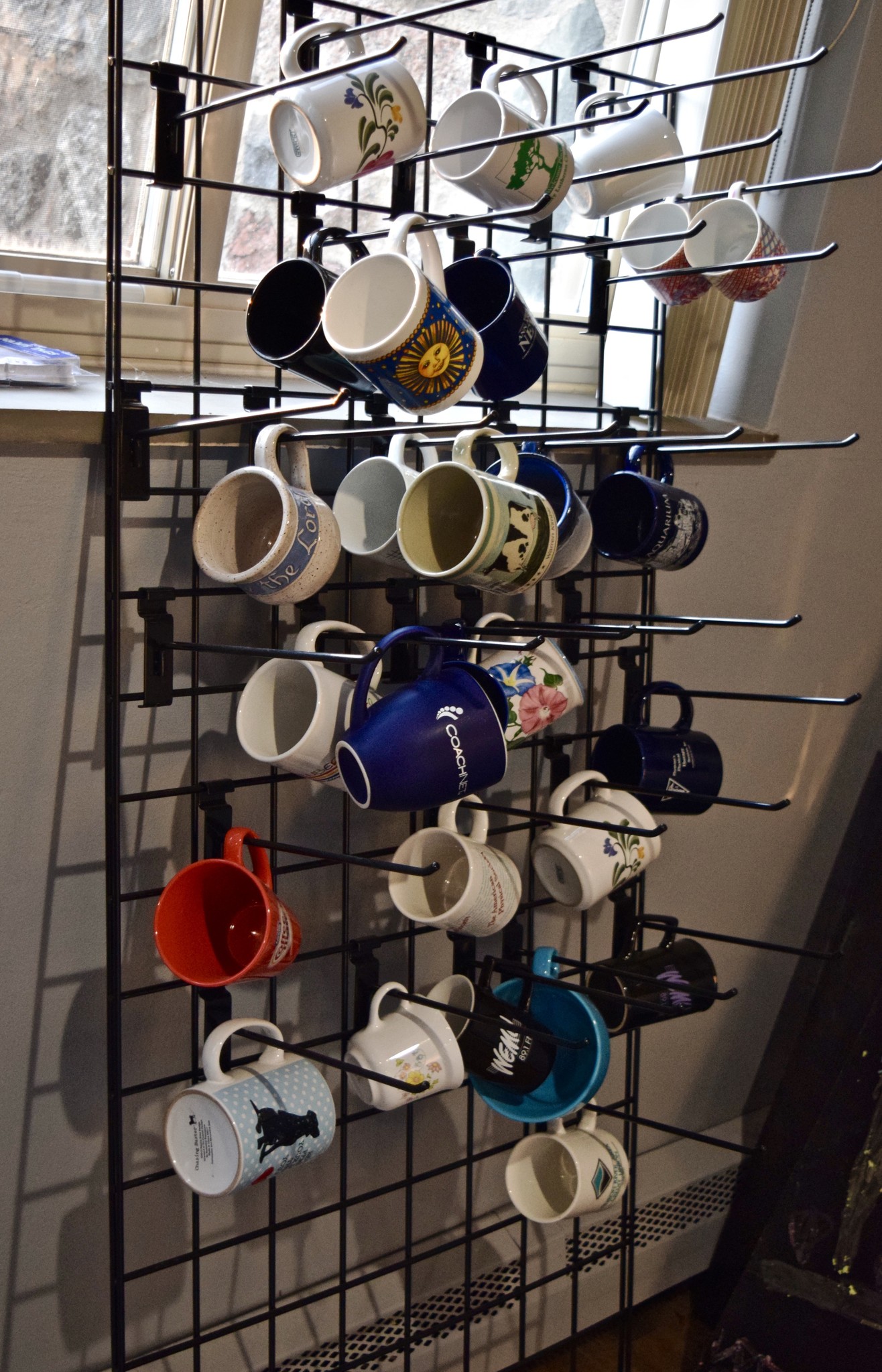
387;793;523;937
586;441;709;571
480;440;593;581
466;611;583;748
429;64;574;224
334;625;507;812
268;22;427;193
153;828;302;988
344;916;719;1223
332;432;440;578
583;680;724;815
620;182;789;306
561;91;686;220
235;621;383;793
245;214;550;417
415;618;509;735
396;426;558;595
165;1019;336;1199
192;423;340;604
530;768;661;912
